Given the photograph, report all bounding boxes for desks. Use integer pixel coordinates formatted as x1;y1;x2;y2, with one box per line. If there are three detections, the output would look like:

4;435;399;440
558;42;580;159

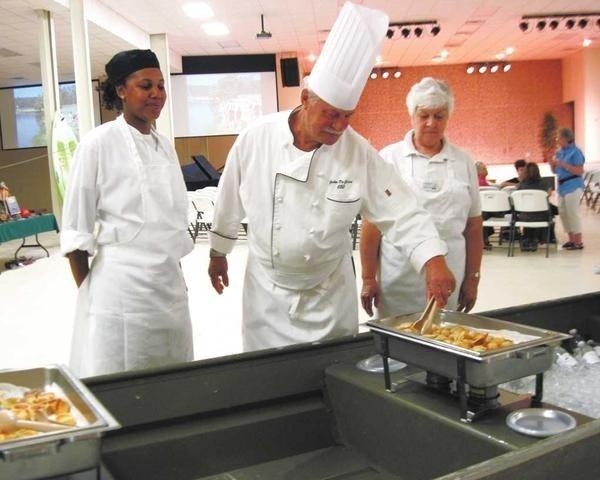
1;212;56;261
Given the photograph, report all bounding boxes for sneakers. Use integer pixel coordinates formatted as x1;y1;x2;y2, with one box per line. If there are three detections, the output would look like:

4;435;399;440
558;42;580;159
563;242;583;251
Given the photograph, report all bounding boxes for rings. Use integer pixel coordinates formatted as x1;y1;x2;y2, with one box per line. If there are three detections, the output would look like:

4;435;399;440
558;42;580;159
448;290;452;294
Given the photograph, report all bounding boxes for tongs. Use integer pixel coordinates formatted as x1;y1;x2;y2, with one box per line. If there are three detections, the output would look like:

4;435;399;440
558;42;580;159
411;293;442;336
0;410;81;433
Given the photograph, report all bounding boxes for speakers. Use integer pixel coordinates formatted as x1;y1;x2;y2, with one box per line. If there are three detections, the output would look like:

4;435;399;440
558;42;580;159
280;58;300;87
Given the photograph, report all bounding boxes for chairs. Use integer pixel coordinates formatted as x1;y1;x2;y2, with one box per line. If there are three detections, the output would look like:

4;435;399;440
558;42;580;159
479;169;600;258
185;186;247;244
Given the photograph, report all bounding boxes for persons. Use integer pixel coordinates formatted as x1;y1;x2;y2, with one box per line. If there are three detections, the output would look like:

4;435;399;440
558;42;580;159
548;127;588;251
474;161;495;250
499;159;527;188
206;75;458;353
515;162;553;252
360;76;484;320
59;47;197;380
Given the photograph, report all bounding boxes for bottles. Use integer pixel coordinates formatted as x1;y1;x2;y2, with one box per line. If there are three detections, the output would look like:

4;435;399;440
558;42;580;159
552;328;600;374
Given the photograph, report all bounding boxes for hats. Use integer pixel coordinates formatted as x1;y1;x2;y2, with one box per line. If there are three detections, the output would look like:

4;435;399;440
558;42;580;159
105;50;160;85
307;2;390;113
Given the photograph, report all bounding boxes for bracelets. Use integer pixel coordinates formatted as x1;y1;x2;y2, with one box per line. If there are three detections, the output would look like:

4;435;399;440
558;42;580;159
465;272;480;278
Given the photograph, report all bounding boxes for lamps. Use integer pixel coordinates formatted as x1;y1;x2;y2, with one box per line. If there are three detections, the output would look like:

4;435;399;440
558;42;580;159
519;11;600;32
386;20;441;39
370;67;401;79
466;63;511;73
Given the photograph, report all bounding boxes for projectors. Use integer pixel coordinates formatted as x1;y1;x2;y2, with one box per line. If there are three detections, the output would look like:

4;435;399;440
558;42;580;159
256;34;272;38
